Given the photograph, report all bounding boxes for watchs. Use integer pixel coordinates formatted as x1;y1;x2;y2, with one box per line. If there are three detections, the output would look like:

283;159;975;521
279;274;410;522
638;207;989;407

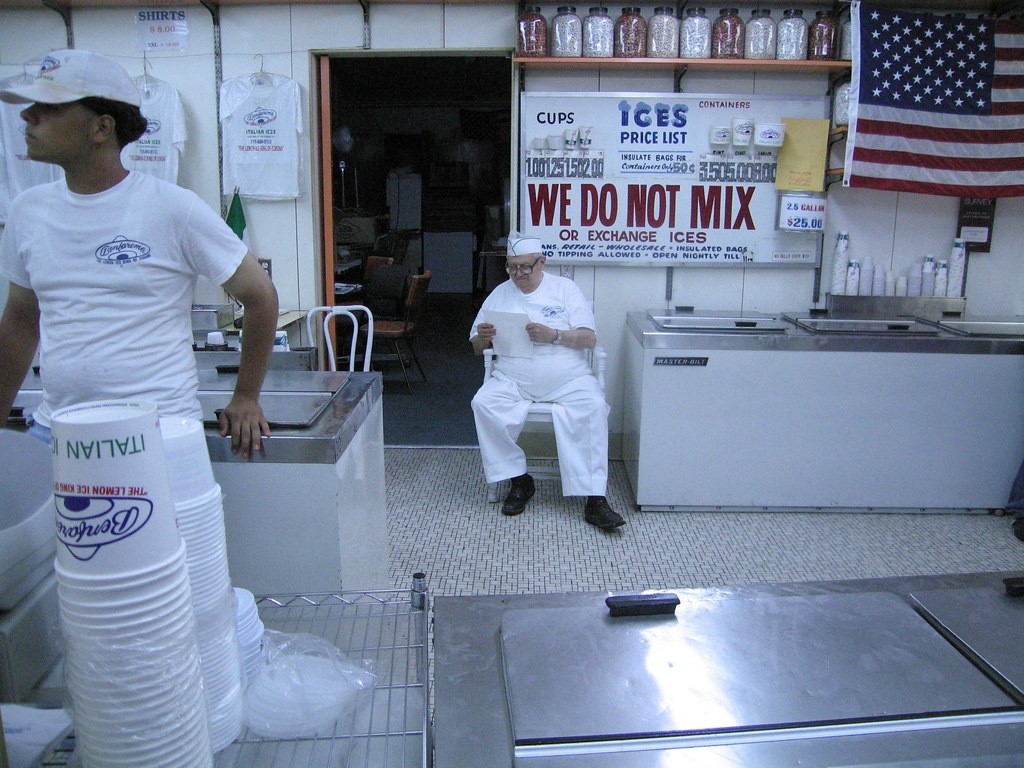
552;329;562;346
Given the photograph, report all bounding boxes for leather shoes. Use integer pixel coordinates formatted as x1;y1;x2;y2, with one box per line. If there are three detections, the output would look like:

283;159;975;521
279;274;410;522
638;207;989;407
585;499;626;529
502;476;535;515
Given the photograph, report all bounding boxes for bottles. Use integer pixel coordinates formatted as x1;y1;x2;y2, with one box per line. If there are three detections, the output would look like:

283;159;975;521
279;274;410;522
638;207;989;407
517;5;853;61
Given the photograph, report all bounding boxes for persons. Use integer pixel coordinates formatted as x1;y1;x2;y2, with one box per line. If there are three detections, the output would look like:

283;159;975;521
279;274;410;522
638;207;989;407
467;230;624;530
2;46;278;457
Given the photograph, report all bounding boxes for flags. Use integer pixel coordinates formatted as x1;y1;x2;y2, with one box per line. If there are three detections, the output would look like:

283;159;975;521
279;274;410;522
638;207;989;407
843;4;1024;201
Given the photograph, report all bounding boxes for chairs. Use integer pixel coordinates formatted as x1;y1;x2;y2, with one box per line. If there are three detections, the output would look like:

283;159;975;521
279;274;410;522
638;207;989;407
477;324;611;503
305;256;435;393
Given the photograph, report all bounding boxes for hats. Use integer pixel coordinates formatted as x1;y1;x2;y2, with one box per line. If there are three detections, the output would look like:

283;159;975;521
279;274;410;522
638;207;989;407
506;230;543;256
0;50;141;106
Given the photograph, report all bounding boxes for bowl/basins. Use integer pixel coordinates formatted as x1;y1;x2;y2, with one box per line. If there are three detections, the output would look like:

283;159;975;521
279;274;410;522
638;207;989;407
754;123;785;146
157;417;264;752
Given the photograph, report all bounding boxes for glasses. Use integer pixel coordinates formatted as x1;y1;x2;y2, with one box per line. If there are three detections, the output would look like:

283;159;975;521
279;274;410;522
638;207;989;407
505;256;541;274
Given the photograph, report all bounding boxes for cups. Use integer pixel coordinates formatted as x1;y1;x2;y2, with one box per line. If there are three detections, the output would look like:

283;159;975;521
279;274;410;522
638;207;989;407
580;126;593;148
535;137;545;149
238;330;242;351
732;118;754;146
273;330;290;352
53;399;210;768
548;135;561;149
207;332;224;344
709;127;731;144
831;230;965;299
566;129;579;149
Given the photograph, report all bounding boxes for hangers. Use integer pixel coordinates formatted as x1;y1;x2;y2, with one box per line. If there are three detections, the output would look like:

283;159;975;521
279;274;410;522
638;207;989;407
119;49;173;104
0;56;43;96
222;52;296;92
